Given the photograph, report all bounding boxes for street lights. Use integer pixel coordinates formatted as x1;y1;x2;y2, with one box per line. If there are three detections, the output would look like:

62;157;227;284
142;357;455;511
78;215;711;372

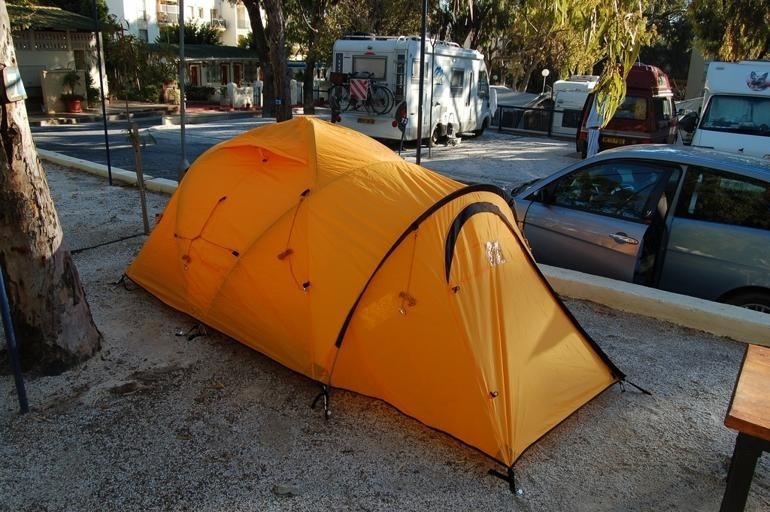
541;68;550;95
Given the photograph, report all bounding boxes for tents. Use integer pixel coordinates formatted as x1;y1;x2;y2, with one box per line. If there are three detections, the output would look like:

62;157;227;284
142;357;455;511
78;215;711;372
107;113;654;495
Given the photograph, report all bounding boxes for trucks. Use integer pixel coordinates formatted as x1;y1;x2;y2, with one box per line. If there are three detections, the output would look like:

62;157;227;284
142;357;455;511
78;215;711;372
330;33;492;148
680;58;770;159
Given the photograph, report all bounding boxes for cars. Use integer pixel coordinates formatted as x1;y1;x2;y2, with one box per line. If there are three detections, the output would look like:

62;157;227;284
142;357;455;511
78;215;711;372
501;143;770;315
488;84;512;95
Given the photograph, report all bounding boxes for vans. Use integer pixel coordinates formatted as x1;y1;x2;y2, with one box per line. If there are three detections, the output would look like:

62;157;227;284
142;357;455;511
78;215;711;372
575;62;683;158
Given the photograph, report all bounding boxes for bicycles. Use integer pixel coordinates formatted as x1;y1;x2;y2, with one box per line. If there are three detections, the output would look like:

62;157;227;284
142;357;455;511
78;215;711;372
328;70;395;115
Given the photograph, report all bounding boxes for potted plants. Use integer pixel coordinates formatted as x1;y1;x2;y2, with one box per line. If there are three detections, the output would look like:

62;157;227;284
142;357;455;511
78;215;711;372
58;70;88;114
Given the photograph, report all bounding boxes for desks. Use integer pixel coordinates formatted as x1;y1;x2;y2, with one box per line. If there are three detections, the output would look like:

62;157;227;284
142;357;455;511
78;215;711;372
721;341;770;512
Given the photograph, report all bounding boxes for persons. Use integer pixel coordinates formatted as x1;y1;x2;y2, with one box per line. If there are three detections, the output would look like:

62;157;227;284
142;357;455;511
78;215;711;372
630;167;669;229
594;168;647;219
